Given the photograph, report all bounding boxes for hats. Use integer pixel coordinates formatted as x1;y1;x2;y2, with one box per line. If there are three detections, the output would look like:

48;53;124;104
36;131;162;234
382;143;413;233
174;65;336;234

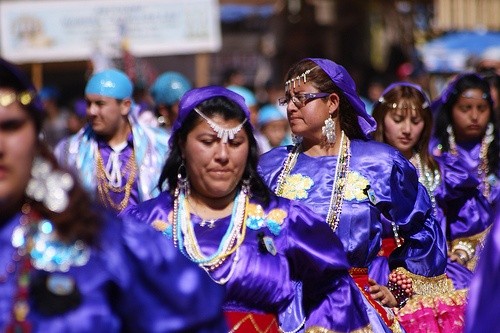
84;69;132;99
227;85;257;108
255;104;285;125
150;72;190;103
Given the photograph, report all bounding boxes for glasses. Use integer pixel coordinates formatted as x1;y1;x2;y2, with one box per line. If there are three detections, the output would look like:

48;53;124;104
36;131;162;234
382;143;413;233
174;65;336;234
278;93;329;108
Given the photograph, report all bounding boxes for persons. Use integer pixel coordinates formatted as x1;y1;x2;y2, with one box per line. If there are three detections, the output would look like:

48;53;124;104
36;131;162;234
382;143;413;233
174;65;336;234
0;58;500;333
